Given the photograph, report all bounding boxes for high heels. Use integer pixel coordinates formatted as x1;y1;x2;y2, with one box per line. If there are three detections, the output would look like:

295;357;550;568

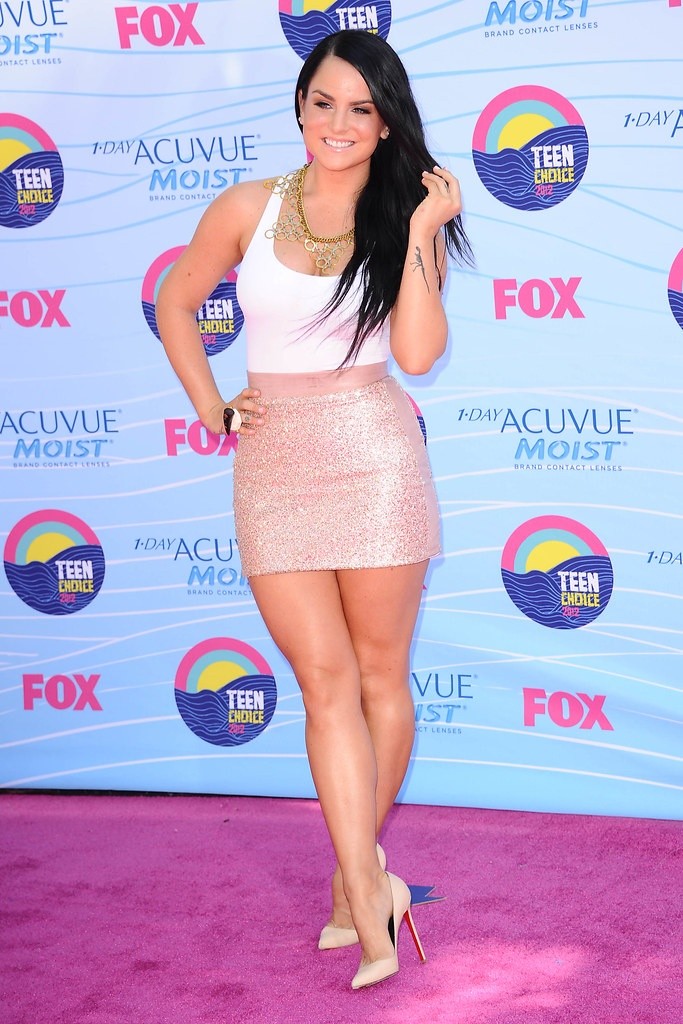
317;841;387;952
349;871;425;990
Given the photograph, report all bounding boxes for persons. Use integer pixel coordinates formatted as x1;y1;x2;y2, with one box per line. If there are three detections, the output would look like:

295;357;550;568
154;29;474;994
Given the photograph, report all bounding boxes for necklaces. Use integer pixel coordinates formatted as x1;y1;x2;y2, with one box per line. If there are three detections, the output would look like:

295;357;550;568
263;159;355;275
